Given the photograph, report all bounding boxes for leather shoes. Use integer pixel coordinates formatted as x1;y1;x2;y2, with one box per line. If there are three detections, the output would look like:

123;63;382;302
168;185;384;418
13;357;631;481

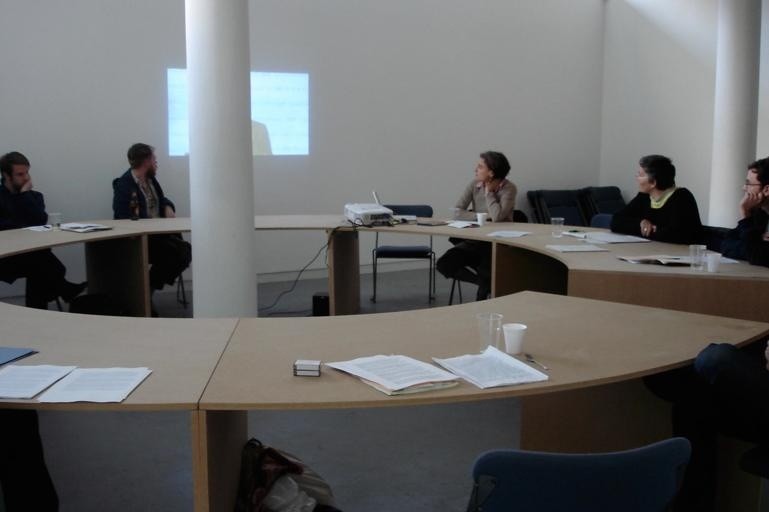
63;281;88;303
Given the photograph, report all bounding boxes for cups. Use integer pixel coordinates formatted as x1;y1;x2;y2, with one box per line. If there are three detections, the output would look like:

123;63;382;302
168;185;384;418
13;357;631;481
690;245;707;273
501;323;527;358
477;311;503;356
477;212;488;226
48;213;62;231
707;253;723;273
551;218;564;238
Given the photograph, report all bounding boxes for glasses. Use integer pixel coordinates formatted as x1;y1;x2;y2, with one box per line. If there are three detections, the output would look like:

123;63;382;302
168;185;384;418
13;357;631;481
744;179;761;185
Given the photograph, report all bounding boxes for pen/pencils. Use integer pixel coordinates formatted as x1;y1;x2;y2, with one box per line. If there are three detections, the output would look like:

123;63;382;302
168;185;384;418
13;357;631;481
43;225;50;229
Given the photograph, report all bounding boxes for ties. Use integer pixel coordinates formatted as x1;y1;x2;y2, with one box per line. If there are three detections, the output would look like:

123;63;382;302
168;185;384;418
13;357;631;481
141;178;155;217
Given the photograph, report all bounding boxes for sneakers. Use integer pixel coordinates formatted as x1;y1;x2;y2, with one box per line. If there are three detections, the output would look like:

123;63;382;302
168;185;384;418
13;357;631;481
476;278;489;301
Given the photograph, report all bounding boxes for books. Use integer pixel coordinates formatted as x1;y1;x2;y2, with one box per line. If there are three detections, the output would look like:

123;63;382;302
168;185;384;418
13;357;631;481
60;223;112;234
616;255;739;266
448;221;480;228
353;371;460;396
562;231;651;244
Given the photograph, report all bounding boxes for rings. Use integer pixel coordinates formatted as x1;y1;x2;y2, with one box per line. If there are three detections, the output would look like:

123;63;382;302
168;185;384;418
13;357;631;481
644;228;647;231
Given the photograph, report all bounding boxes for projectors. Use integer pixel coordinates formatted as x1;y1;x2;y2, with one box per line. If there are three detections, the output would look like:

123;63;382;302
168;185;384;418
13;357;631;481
341;191;397;225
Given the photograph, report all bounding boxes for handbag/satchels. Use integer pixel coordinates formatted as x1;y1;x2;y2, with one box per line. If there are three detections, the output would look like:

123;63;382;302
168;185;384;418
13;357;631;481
241;437;334;512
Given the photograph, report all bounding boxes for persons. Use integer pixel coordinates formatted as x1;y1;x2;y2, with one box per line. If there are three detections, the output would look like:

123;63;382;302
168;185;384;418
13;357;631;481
0;151;90;309
610;155;703;246
640;334;769;511
721;158;769;268
435;150;519;302
113;143;192;318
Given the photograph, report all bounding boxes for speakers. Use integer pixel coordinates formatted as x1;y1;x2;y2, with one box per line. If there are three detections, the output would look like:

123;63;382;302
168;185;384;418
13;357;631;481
312;290;329;316
